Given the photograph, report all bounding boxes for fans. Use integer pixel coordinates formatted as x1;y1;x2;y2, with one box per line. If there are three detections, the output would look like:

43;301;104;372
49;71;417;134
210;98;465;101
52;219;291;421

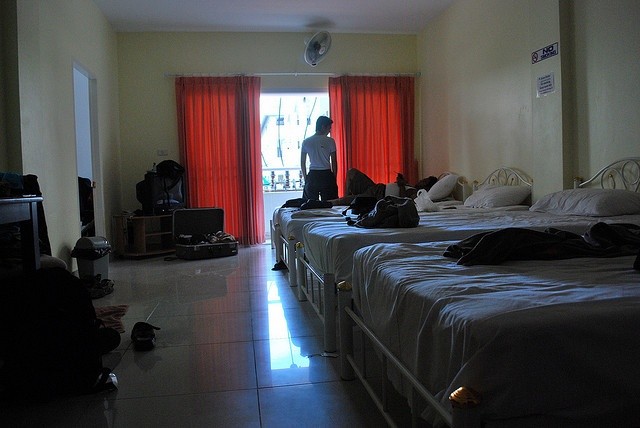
301;28;332;68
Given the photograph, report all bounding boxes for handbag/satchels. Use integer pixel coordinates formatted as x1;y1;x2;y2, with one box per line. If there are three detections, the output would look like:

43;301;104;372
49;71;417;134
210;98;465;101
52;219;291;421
348;196;420;229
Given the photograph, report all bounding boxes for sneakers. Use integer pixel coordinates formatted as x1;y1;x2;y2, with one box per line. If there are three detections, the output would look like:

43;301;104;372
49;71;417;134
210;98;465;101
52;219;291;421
130;322;160;351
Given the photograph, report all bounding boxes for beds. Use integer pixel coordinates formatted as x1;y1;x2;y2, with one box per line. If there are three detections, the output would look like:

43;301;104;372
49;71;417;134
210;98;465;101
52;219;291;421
275;167;535;289
265;159;472;268
295;157;640;363
335;230;640;427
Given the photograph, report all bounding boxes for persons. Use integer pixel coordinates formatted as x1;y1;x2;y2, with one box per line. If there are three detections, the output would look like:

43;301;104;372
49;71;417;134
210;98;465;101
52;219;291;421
300;116;338;200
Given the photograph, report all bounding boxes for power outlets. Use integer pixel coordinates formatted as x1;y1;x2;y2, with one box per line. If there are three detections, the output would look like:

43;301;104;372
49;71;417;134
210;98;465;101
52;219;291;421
157;148;169;155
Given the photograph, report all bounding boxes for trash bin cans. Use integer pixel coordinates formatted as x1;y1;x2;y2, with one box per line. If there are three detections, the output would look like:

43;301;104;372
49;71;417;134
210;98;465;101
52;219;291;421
76;236;111;284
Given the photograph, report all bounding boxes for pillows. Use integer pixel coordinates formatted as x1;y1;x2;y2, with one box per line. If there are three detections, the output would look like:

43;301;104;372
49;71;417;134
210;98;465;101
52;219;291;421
462;185;530;208
529;189;640;217
422;174;459;199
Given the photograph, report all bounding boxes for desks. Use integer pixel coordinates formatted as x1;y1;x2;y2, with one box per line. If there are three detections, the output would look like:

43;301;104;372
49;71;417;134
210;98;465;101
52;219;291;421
0;195;43;276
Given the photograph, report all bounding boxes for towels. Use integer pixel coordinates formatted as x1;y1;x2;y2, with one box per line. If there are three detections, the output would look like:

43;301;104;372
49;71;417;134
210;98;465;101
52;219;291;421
94;304;129;334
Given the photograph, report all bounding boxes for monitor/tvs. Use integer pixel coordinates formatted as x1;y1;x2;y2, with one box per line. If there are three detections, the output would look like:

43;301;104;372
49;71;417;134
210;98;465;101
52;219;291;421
136;160;187;216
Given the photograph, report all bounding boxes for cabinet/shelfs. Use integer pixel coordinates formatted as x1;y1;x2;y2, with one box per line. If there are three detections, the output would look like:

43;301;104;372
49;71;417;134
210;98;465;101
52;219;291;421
112;214;174;257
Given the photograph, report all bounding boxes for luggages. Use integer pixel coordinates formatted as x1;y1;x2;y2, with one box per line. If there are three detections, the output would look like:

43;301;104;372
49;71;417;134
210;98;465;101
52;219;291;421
172;207;239;261
